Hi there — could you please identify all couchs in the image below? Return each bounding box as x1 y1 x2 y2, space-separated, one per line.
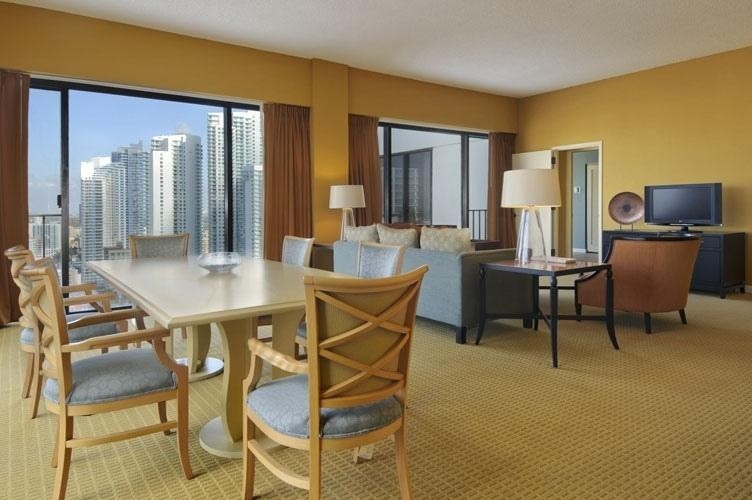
573 230 703 335
333 235 534 343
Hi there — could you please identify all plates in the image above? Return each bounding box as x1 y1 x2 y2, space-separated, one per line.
608 192 644 225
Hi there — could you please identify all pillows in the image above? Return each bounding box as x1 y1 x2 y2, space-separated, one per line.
343 225 379 241
418 226 470 251
377 223 418 247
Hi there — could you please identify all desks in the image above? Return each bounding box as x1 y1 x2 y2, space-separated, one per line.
475 258 621 370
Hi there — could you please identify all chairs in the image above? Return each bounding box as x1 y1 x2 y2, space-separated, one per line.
127 233 191 343
252 234 316 360
5 242 145 422
291 240 408 361
238 262 429 500
32 259 193 500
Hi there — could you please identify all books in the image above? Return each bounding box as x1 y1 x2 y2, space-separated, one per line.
530 254 577 265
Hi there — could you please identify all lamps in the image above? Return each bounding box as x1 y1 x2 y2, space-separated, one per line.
327 184 367 238
499 169 562 263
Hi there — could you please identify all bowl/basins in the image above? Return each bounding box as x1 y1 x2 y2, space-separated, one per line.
197 252 241 273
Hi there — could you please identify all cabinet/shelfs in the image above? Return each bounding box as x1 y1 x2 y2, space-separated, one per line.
602 228 746 298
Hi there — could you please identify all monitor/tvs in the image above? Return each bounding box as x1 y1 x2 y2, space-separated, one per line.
643 183 722 233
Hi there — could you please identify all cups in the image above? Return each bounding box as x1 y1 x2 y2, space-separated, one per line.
524 248 532 263
